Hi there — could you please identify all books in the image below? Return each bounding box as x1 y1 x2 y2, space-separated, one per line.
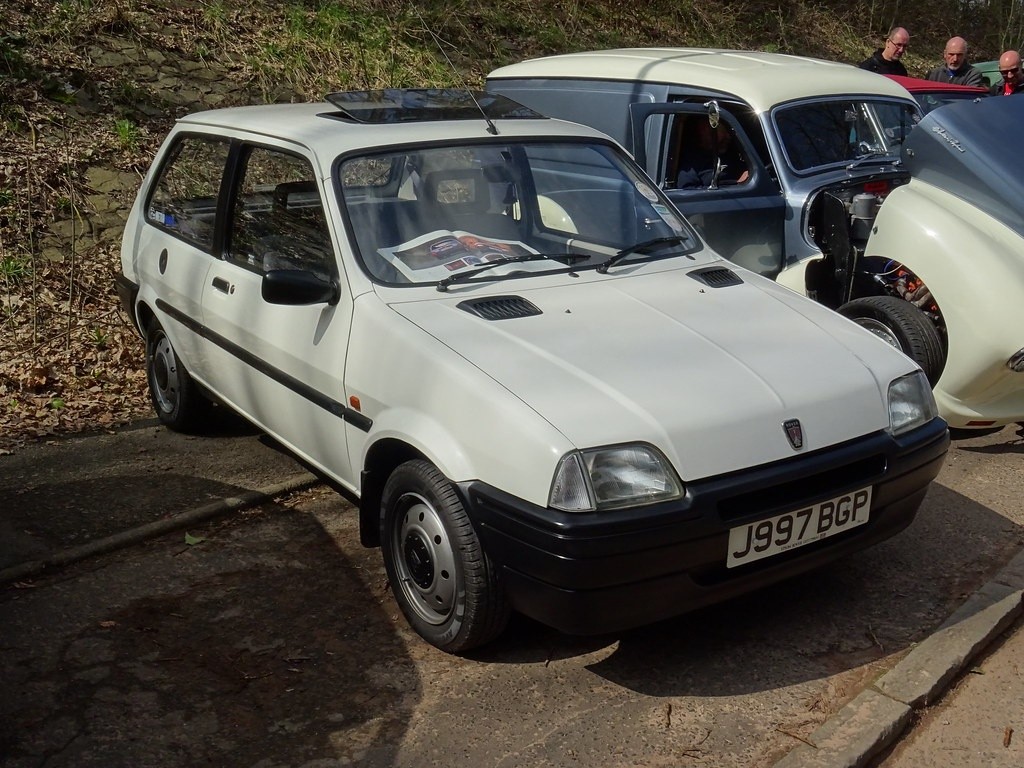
377 229 572 283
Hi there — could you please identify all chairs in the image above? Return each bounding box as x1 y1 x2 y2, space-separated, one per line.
421 168 522 247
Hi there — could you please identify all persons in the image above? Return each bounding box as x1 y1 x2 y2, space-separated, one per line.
778 105 874 170
854 27 910 77
665 104 774 189
917 36 985 117
989 50 1024 96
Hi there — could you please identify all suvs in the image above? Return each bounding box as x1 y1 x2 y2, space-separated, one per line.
122 87 952 655
482 49 1024 431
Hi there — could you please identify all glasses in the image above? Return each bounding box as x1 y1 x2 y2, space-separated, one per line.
888 38 909 50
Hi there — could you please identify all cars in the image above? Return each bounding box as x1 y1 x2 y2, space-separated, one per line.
853 75 994 194
